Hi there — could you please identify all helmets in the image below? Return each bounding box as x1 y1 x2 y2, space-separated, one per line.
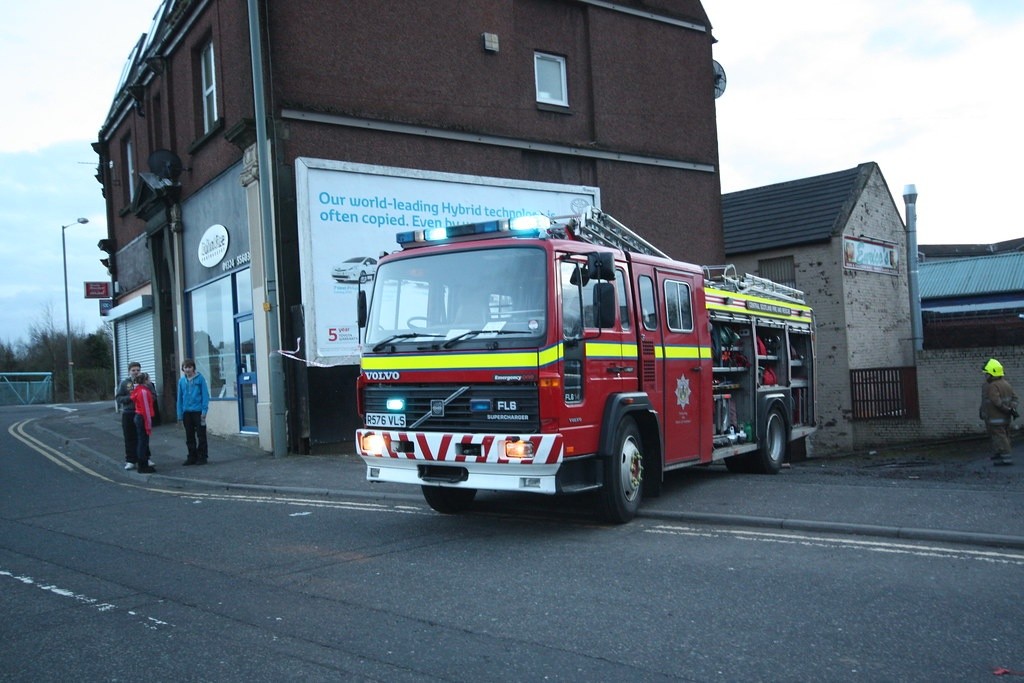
983 359 1006 377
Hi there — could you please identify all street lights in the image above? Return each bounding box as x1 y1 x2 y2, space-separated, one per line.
61 217 91 402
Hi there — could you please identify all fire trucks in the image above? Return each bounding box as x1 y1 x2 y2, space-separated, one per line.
350 203 820 526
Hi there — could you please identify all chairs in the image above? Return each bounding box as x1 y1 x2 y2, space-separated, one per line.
454 280 491 327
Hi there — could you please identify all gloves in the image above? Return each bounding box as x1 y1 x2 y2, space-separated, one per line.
1010 408 1020 420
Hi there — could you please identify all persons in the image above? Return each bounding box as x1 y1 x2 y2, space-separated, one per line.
979 357 1020 466
131 371 158 473
177 358 211 466
114 361 156 470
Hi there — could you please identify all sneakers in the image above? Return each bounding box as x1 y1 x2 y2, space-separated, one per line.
147 460 156 467
124 463 136 469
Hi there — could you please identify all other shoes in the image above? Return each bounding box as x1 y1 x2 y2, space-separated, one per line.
196 459 207 464
138 466 155 473
991 454 1001 460
183 460 198 465
993 460 1012 465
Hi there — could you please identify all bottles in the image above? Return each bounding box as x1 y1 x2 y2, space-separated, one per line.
201 418 205 426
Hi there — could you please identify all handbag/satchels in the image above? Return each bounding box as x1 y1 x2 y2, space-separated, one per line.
1008 415 1021 430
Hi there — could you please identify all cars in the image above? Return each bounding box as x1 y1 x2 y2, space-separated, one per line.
330 256 380 284
